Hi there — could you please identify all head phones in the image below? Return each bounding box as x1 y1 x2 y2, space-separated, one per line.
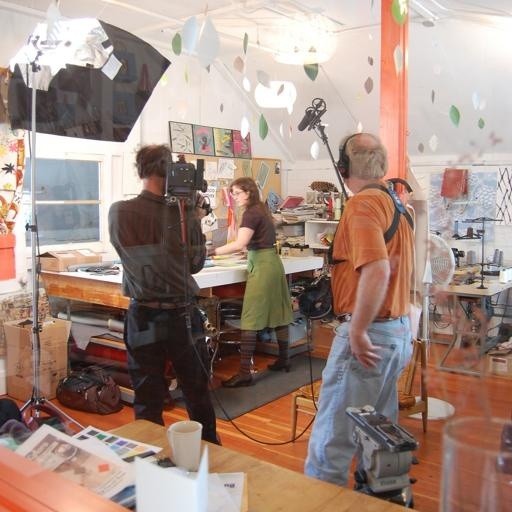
338 133 360 178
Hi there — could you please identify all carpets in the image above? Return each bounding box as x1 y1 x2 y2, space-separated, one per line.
170 354 332 424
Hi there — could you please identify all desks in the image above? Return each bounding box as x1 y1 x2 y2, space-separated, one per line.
436 266 510 383
39 253 325 403
103 414 415 511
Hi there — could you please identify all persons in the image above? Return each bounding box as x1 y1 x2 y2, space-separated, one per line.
204 177 294 388
108 144 227 449
301 132 414 490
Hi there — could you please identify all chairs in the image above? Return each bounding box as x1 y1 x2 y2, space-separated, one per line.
289 336 431 449
206 299 243 379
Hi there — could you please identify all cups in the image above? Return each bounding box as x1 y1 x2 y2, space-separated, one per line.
439 416 512 511
168 421 203 472
465 250 476 265
492 248 503 267
467 227 473 238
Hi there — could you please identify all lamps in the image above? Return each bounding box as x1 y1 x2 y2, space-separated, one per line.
5 14 171 436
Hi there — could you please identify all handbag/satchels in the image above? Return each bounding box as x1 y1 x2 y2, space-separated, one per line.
55 364 124 416
298 273 334 320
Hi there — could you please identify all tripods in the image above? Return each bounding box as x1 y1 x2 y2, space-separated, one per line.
20 122 83 432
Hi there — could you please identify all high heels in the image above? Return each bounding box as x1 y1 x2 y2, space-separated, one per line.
221 371 257 388
266 360 292 373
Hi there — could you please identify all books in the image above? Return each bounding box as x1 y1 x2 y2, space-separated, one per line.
278 194 304 209
277 204 329 247
16 416 168 509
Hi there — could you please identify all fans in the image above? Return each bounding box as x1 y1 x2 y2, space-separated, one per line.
411 229 461 423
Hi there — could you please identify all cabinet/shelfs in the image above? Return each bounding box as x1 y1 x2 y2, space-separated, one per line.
305 220 338 249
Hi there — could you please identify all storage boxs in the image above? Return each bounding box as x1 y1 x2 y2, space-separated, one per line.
279 223 304 238
3 316 73 406
485 346 512 379
36 247 103 273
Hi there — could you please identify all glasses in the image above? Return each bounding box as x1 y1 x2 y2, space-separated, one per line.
231 189 244 196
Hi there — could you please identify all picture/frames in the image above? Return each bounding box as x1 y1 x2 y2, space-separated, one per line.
168 120 254 160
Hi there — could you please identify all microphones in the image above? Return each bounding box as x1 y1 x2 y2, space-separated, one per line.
298 111 316 131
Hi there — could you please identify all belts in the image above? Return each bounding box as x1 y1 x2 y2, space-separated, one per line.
129 297 191 310
337 311 399 323
